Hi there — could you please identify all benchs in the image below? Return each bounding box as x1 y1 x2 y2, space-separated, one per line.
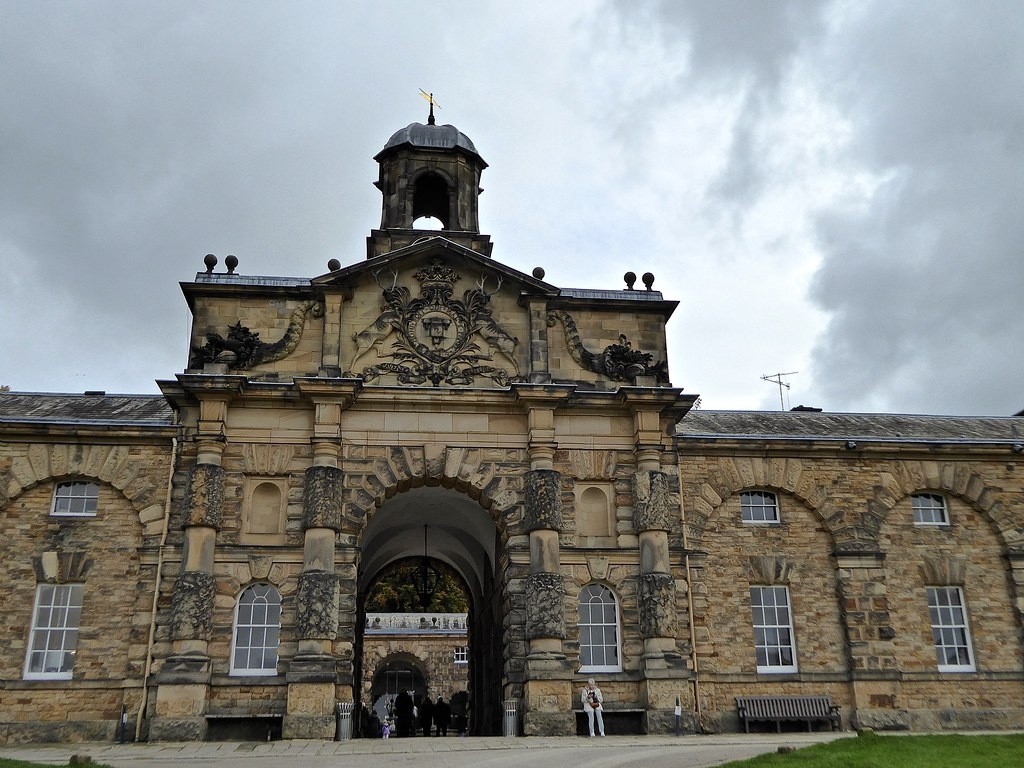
734 695 843 733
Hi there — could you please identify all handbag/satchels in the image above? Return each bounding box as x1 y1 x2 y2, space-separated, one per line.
446 715 451 724
416 718 420 726
587 692 599 708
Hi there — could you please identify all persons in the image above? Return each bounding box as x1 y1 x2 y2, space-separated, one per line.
382 715 391 738
581 678 605 737
419 697 435 737
395 688 414 738
433 696 451 737
369 709 381 737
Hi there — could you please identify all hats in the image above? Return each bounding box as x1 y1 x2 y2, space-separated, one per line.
372 710 377 714
398 688 406 693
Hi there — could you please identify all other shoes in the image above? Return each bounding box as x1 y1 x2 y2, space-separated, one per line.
591 733 595 737
601 731 605 737
